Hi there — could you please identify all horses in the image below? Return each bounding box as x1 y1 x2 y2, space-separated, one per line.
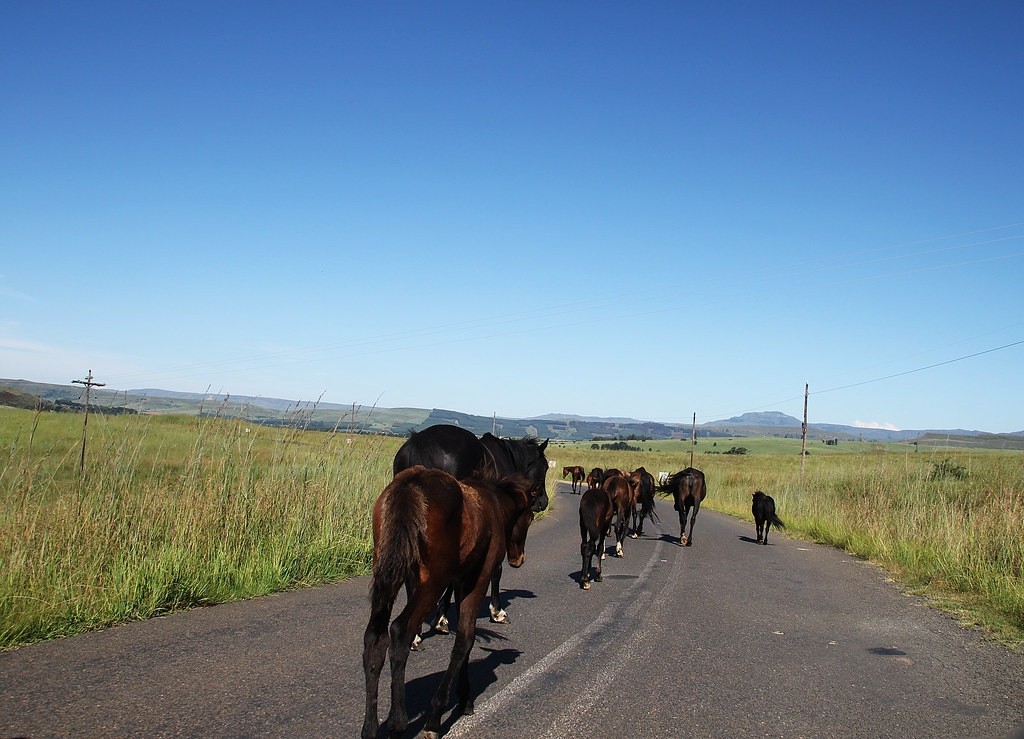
562 466 661 590
655 467 706 547
361 424 553 739
751 491 787 546
